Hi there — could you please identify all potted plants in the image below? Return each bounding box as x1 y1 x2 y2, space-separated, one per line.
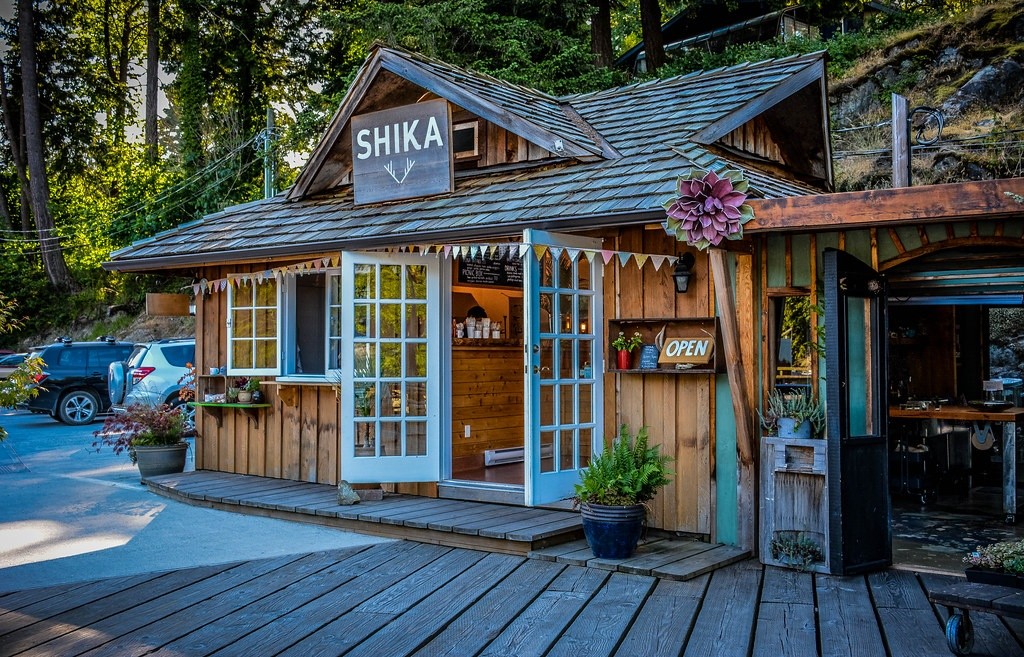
753 386 826 438
134 441 190 484
247 379 266 404
327 352 390 490
573 425 677 559
236 377 252 403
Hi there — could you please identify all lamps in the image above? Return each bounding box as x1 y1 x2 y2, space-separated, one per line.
484 444 553 466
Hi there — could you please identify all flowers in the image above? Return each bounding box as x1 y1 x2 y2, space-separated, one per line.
91 403 199 466
612 331 643 353
660 168 755 251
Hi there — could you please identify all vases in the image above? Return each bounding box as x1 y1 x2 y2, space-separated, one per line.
617 349 631 370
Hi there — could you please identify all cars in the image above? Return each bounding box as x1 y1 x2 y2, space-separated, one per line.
0 352 32 380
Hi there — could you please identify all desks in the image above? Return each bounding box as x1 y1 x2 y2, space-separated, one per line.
889 403 1023 523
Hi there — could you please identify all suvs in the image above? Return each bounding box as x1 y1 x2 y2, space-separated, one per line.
23 335 134 424
107 336 195 437
